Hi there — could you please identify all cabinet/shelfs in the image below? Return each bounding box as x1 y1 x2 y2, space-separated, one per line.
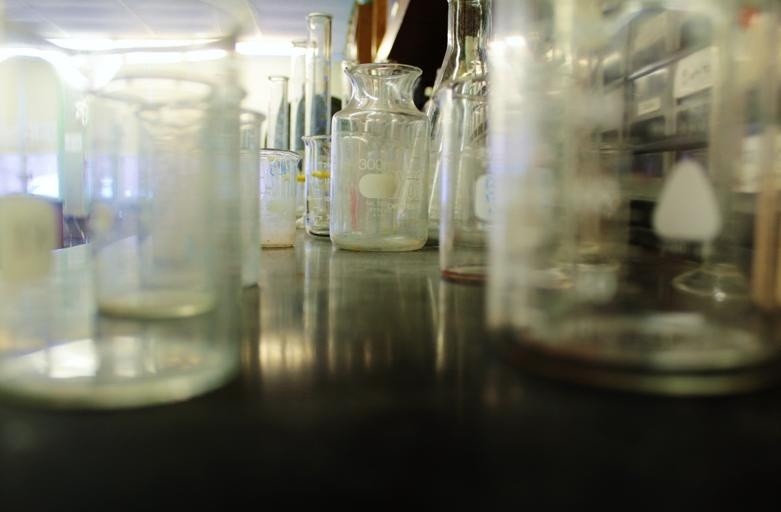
329 60 432 252
390 0 488 244
302 13 334 239
266 75 289 152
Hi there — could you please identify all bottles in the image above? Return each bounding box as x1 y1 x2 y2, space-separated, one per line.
329 60 432 252
390 0 488 244
302 13 334 239
483 1 779 399
266 75 289 152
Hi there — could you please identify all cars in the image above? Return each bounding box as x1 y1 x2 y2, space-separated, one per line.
0 0 260 412
129 105 267 292
299 134 371 235
440 89 575 288
236 148 304 249
79 73 225 322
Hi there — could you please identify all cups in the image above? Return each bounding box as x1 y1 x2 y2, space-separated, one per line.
440 89 575 288
129 105 267 292
236 148 304 249
299 134 371 235
0 0 260 412
79 73 225 322
